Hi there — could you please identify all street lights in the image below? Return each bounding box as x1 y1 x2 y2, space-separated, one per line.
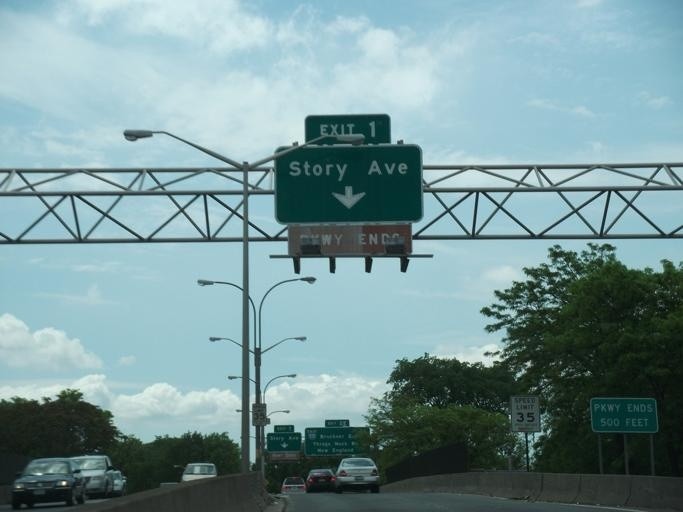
233 408 291 466
228 374 298 462
196 276 318 471
122 129 367 472
240 435 266 463
209 335 307 475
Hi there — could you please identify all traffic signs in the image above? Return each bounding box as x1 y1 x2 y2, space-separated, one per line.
591 397 658 433
275 147 423 222
274 425 293 432
265 450 300 462
288 223 412 256
511 396 540 432
305 427 370 457
305 115 390 144
267 433 301 451
324 419 349 428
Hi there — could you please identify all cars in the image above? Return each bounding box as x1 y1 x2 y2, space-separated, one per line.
180 462 218 483
280 476 306 495
304 468 334 491
70 453 115 499
7 457 86 510
113 470 127 497
334 458 381 493
